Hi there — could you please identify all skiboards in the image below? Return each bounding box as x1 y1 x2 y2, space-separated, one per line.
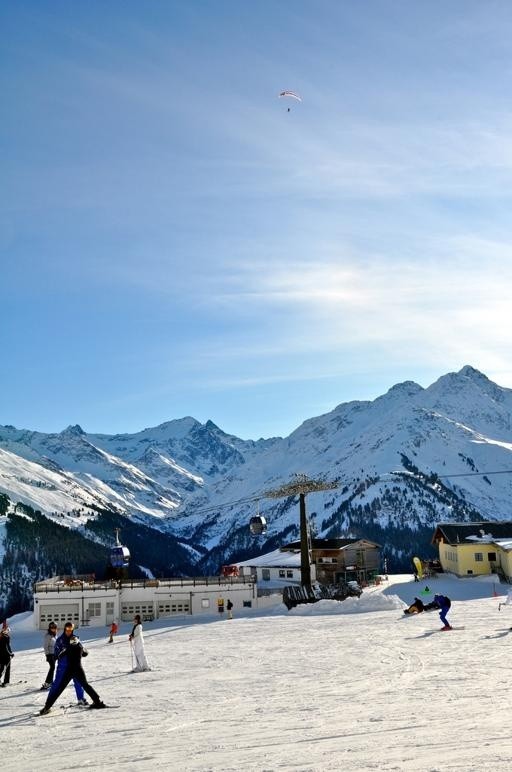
113 670 160 674
28 683 120 717
1 681 27 686
426 626 464 631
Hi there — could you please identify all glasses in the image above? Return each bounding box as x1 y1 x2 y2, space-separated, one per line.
48 624 57 628
65 627 73 631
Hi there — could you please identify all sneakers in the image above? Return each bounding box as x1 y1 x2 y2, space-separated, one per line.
41 682 53 689
39 708 49 714
442 625 452 631
78 699 88 706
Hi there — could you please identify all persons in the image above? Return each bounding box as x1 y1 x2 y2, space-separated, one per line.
109 620 118 643
403 593 453 630
503 587 512 630
216 595 234 619
0 624 14 688
39 621 108 714
129 614 151 672
414 569 419 582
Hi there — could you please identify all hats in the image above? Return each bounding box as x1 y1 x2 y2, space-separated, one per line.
2 626 11 634
134 615 140 621
